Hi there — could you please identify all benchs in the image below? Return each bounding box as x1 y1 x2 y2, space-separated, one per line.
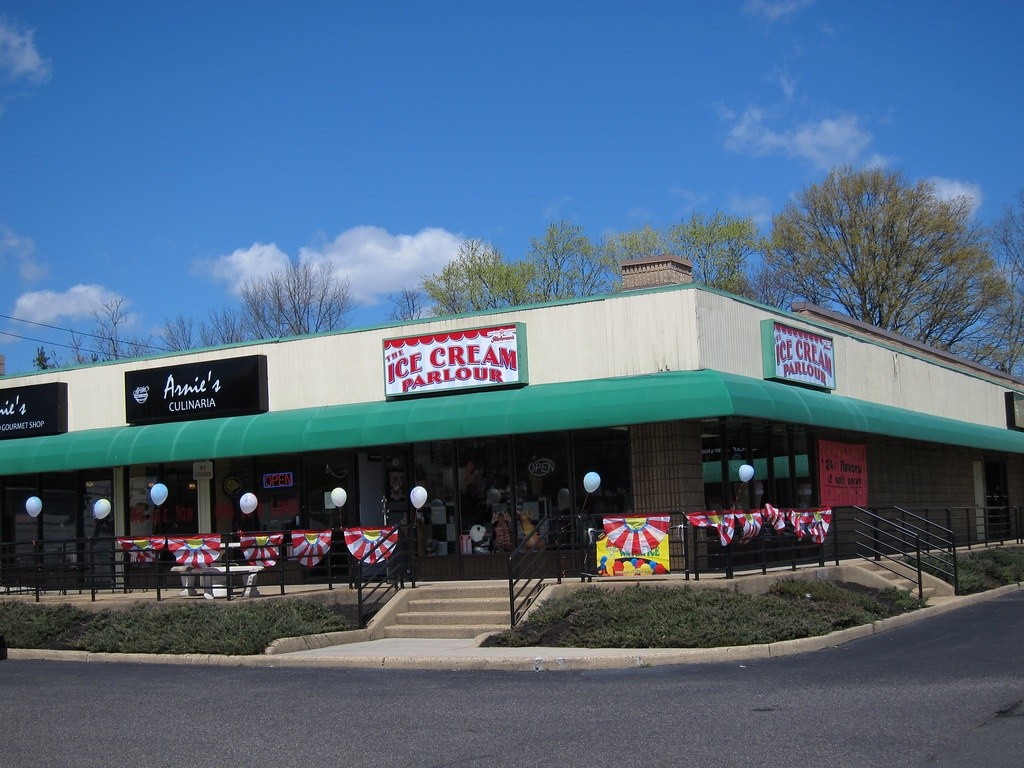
170 566 266 598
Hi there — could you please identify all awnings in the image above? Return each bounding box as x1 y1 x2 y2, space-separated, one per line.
0 368 1024 475
